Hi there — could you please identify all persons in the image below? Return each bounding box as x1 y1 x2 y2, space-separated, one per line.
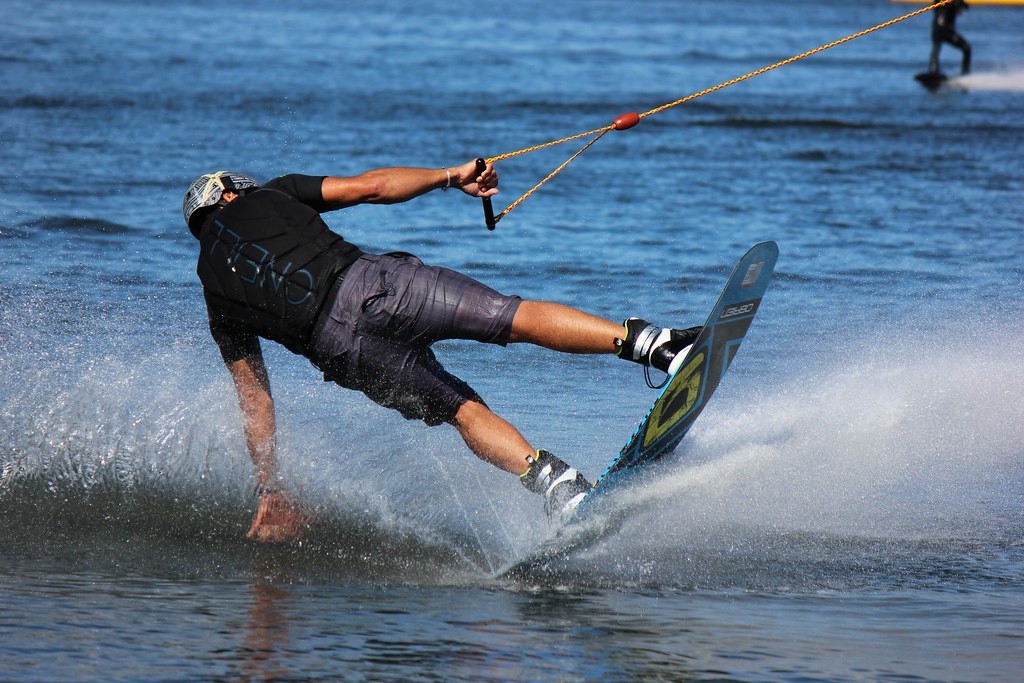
928 0 973 76
179 158 705 548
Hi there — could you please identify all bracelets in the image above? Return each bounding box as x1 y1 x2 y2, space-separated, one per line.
440 167 450 192
254 485 281 498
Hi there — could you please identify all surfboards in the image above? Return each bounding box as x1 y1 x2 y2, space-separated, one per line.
914 72 969 95
493 240 780 579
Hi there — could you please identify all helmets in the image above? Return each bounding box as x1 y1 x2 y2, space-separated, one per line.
181 172 260 239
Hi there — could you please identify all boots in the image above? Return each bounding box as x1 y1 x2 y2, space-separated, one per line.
519 449 594 539
615 316 701 376
